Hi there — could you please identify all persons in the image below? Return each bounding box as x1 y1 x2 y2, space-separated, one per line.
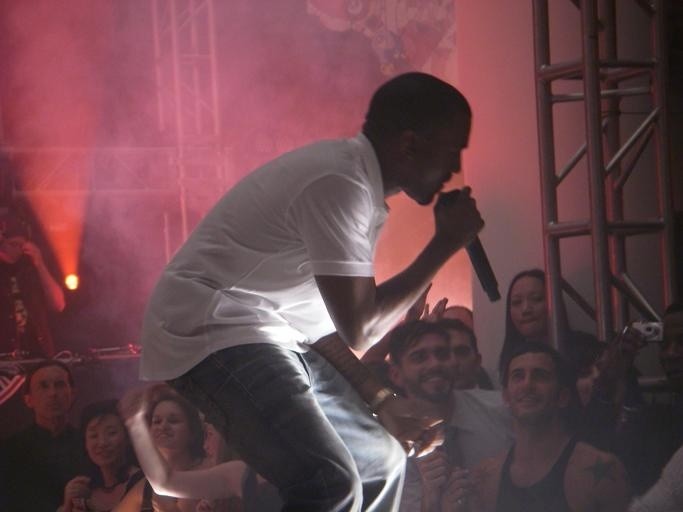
136 73 472 512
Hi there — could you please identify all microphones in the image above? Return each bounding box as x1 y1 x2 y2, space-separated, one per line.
443 190 500 304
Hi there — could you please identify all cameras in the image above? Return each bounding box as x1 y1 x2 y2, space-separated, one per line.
631 321 663 343
